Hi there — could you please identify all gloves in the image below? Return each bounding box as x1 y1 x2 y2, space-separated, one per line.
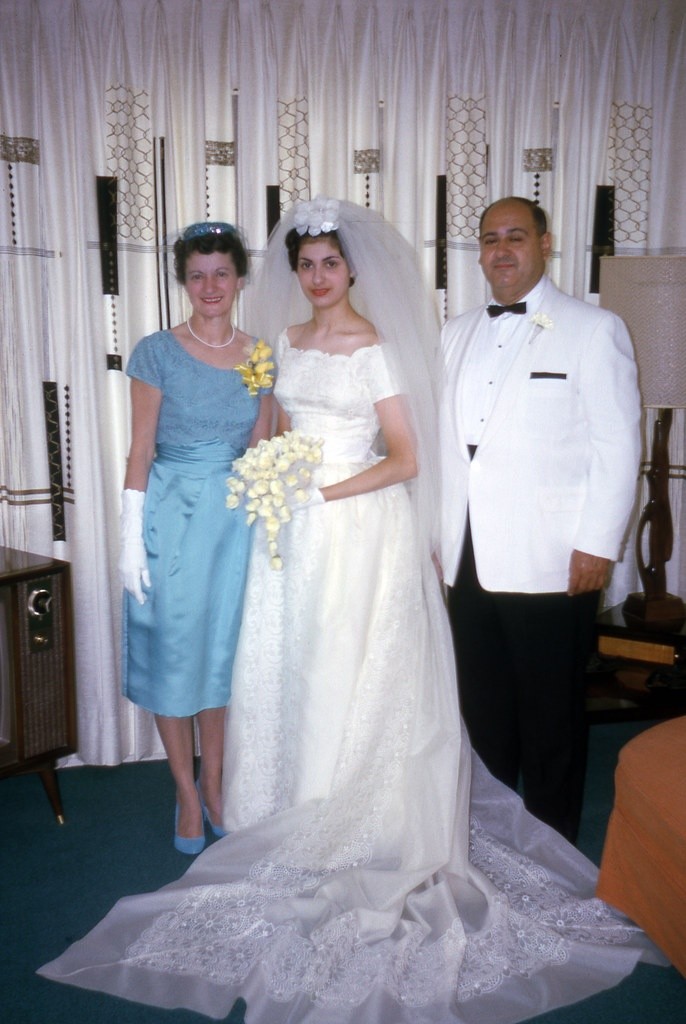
116 489 152 605
289 487 325 510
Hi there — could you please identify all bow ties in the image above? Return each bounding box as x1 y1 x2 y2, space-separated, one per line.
487 302 526 318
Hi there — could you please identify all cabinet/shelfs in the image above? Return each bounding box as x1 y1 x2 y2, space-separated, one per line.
0 545 78 826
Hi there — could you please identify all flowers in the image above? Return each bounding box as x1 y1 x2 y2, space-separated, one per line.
225 430 324 572
233 339 274 398
294 198 341 237
530 313 553 331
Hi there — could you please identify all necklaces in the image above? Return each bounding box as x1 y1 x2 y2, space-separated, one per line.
185 315 235 347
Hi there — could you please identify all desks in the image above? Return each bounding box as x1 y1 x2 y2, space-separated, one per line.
584 600 686 725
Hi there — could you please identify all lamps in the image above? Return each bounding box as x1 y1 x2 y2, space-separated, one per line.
600 255 686 629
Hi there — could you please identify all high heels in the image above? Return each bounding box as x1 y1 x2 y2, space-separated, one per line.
196 779 226 838
174 801 205 855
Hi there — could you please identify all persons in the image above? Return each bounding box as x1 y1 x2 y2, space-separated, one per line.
118 196 644 869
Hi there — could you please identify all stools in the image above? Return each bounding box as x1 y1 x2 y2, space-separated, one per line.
595 716 686 980
